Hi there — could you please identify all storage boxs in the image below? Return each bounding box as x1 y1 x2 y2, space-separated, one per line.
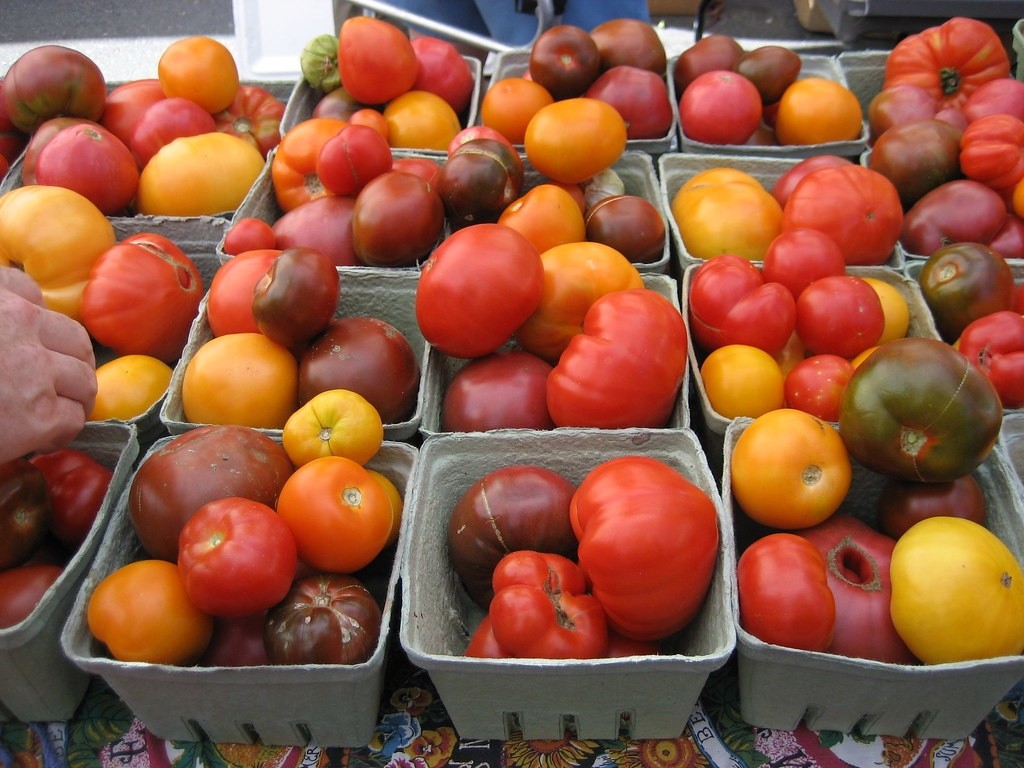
161 269 431 439
413 272 691 434
998 411 1024 500
0 80 296 218
0 422 139 722
95 215 231 469
278 57 482 157
659 151 904 284
856 147 1024 267
398 427 738 742
681 264 945 489
474 50 678 177
904 258 1024 417
441 149 671 274
59 435 417 749
722 416 1024 742
665 53 870 164
212 150 449 272
834 48 1014 150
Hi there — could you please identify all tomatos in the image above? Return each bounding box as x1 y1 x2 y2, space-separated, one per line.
1 7 1024 676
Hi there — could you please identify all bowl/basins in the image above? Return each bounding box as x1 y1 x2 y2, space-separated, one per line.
1 48 1024 745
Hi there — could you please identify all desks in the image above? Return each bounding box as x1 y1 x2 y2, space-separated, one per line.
0 647 1024 768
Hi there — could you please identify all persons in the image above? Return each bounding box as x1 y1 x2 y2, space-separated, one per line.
0 265 99 469
